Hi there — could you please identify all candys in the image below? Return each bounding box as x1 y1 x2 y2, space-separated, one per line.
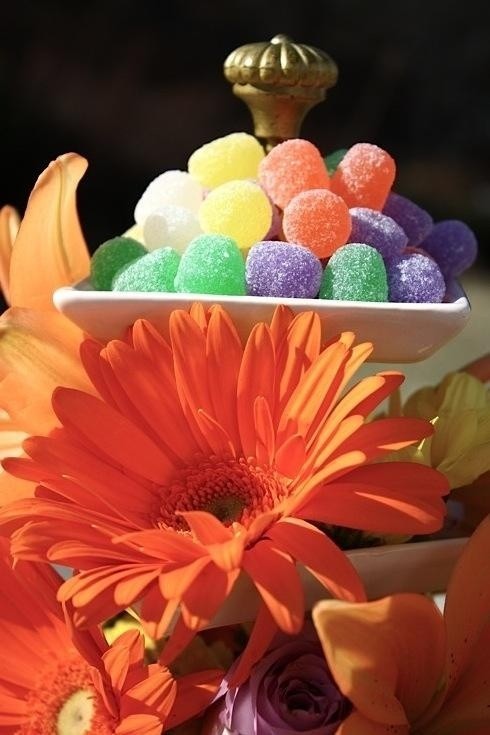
89 131 479 303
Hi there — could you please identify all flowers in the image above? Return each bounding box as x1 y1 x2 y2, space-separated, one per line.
0 146 490 735
